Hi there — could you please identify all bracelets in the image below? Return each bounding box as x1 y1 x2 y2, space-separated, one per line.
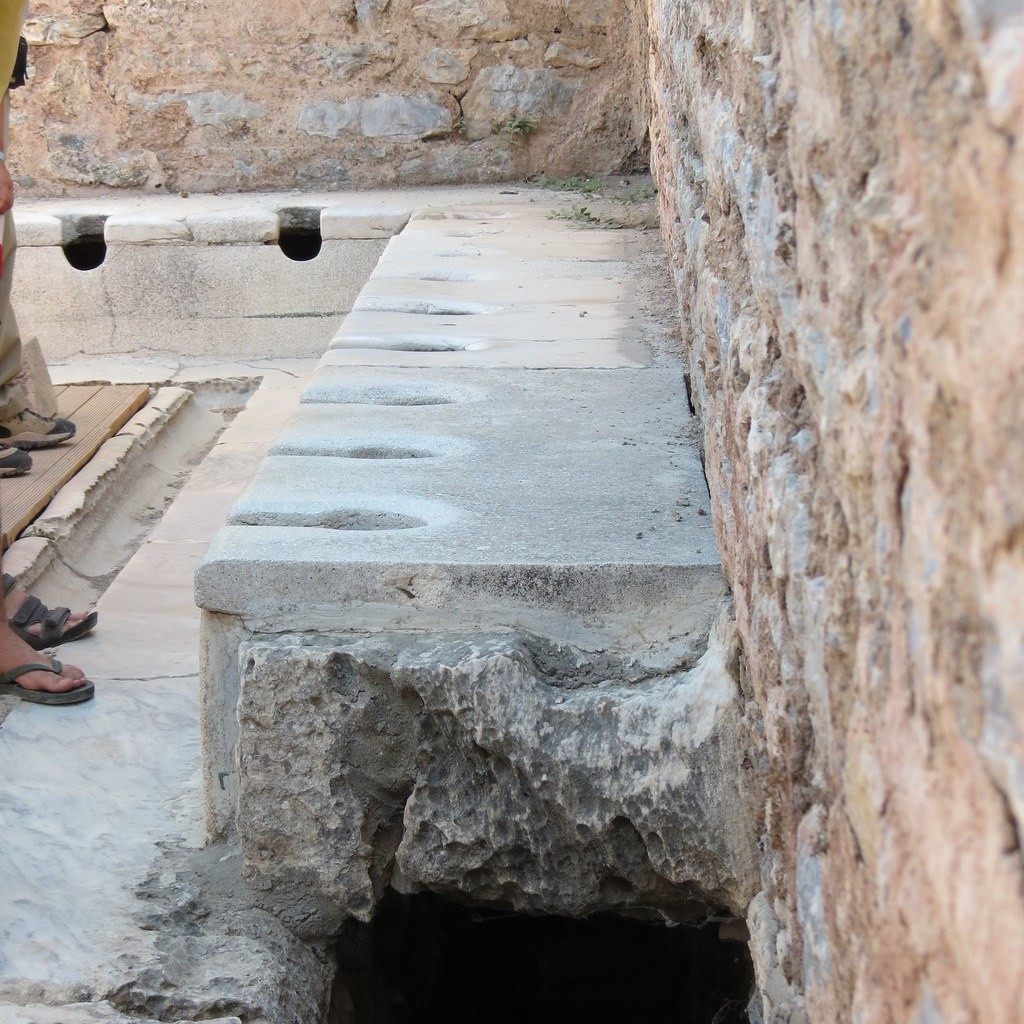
0 152 6 161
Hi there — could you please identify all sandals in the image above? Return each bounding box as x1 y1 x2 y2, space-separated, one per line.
0 575 98 651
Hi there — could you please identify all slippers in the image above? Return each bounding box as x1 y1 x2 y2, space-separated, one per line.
0 655 94 705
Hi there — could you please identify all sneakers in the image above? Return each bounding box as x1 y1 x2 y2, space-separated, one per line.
0 406 77 449
0 442 33 478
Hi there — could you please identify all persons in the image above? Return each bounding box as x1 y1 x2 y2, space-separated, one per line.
0 570 98 704
0 1 77 478
